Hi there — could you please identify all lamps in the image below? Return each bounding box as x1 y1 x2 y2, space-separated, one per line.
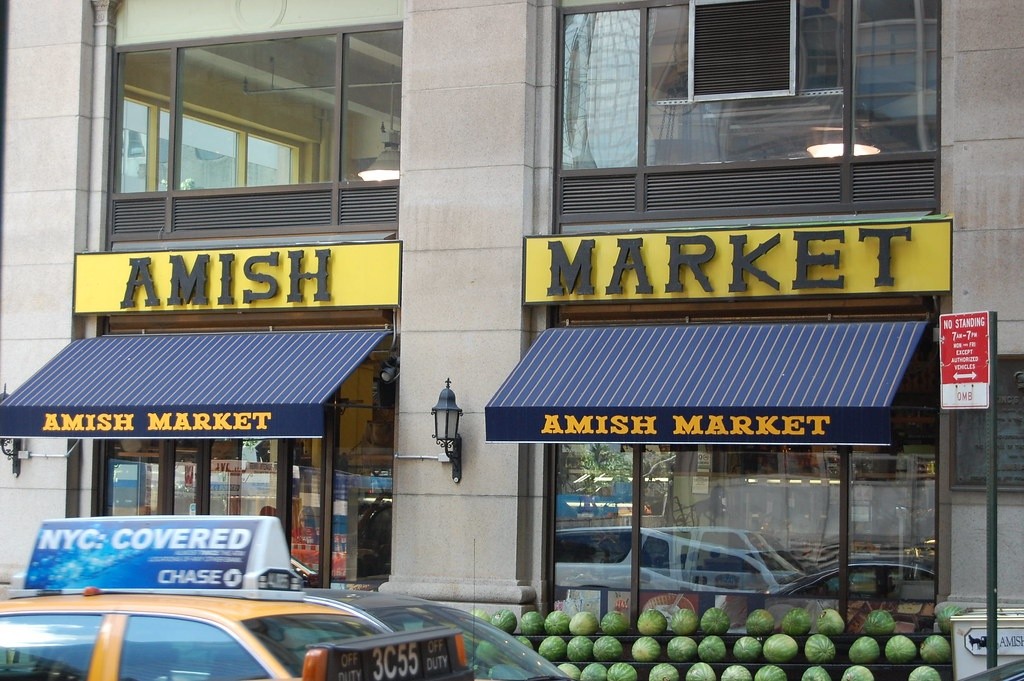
430 377 463 483
357 85 400 180
380 354 400 383
0 383 21 478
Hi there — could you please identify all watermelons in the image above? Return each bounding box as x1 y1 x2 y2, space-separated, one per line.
451 606 966 681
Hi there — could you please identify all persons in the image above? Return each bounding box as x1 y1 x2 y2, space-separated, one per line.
259 506 276 516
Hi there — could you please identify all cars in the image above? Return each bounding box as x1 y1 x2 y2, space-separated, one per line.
0 515 577 681
553 524 937 598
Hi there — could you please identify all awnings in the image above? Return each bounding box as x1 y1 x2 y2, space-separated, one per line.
0 328 390 439
485 320 935 448
